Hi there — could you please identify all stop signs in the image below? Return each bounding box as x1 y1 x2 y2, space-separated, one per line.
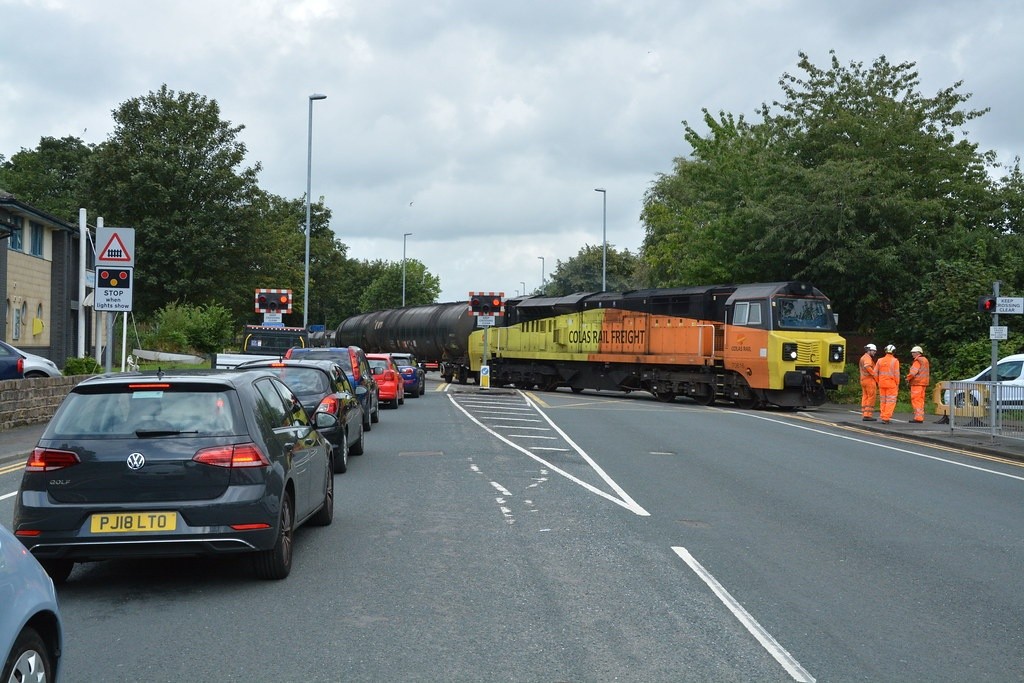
95 266 133 313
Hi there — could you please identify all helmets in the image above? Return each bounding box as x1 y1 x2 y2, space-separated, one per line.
910 346 923 353
864 344 877 351
884 344 896 353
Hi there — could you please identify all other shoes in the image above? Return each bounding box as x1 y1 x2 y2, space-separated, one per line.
909 419 923 423
881 419 893 424
862 416 877 421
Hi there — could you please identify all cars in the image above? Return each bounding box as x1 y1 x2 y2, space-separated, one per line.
944 354 1024 410
363 352 406 409
392 353 425 398
0 368 337 683
0 340 62 381
235 360 368 474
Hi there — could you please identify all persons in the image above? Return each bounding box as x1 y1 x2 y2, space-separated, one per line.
859 344 877 421
873 344 900 424
905 346 929 423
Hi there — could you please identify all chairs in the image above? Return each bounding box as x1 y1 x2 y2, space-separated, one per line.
131 398 174 430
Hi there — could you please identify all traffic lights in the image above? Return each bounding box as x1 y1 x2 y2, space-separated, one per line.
255 288 292 314
978 296 996 314
468 291 505 317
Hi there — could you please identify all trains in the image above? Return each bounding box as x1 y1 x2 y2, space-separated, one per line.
308 281 850 409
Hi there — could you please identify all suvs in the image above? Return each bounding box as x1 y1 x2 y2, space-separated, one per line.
284 346 384 431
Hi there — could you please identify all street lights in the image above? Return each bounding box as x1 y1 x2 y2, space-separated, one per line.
402 233 412 307
538 256 544 295
595 187 607 293
515 282 525 298
304 94 327 328
